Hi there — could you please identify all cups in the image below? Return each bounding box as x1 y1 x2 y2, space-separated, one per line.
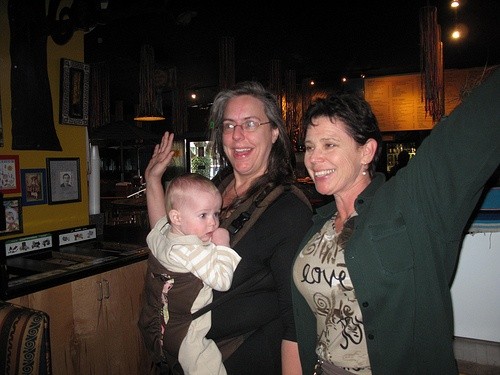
90 147 100 214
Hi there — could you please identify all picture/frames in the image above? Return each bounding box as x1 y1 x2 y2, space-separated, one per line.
0 156 22 194
21 168 48 205
0 196 24 236
46 157 81 205
60 58 91 127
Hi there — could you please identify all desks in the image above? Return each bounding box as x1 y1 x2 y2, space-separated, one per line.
101 182 148 235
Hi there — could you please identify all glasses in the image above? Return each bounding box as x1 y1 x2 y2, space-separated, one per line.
219 121 273 133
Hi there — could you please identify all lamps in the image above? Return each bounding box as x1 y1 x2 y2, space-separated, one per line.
134 84 166 122
46 1 110 46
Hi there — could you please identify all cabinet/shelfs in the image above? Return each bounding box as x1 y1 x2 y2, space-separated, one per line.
0 226 147 375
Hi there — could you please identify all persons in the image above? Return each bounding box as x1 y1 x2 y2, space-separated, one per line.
61 172 70 187
292 67 500 375
136 173 243 375
145 86 313 375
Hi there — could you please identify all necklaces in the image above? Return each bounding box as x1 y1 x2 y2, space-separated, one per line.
233 179 239 197
